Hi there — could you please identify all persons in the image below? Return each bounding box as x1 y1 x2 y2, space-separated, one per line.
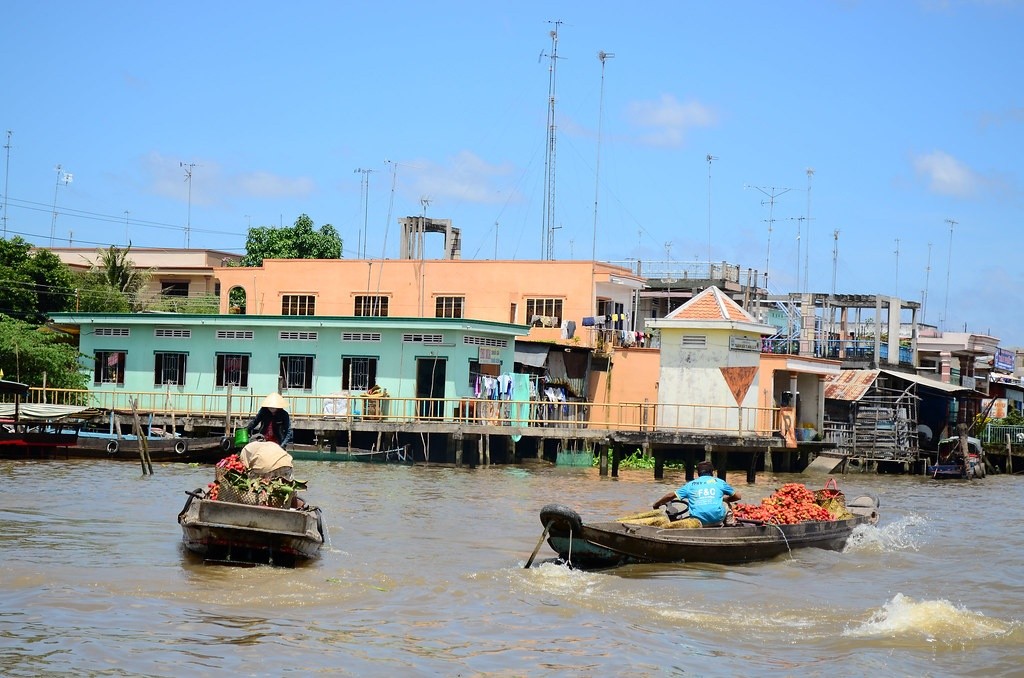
247 392 292 449
238 434 298 508
652 461 741 527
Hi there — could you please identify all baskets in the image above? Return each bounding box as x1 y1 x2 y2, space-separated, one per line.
814 478 846 506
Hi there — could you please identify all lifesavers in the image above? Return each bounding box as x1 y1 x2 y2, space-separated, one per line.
106 439 120 454
185 488 202 509
220 437 233 453
540 503 583 534
174 439 188 455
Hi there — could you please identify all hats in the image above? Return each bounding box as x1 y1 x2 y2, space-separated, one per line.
697 461 715 471
261 392 289 408
250 433 266 442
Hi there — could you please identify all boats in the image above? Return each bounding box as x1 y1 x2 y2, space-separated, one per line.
178 489 323 569
930 436 984 480
285 443 404 461
539 494 879 568
0 403 235 457
